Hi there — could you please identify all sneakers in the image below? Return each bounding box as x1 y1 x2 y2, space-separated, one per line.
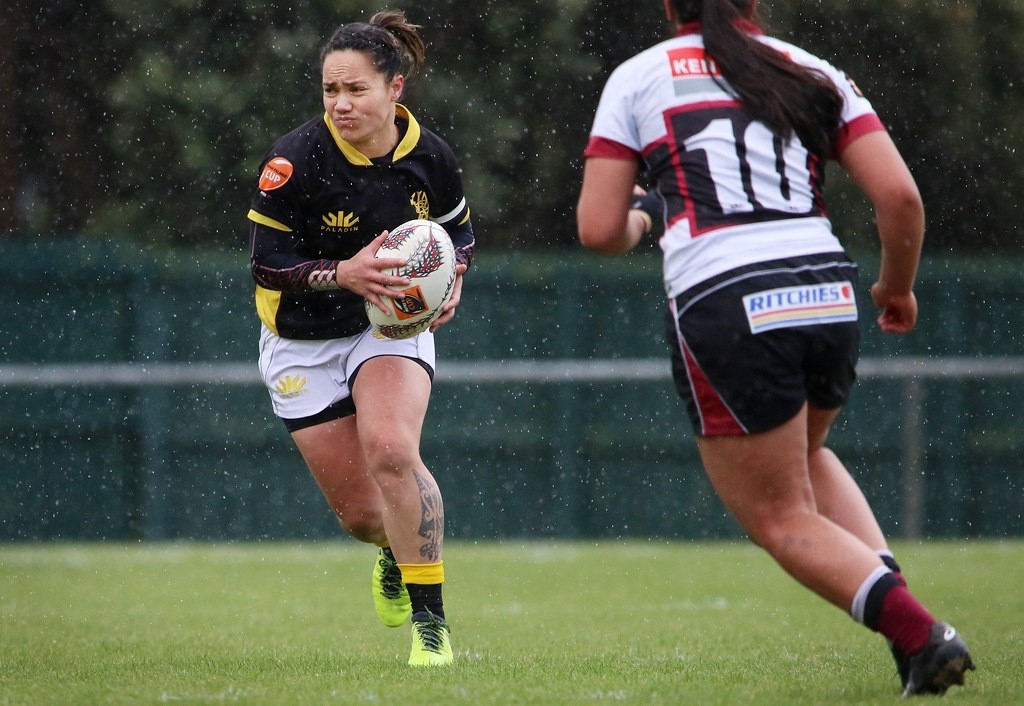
372 547 412 627
408 605 453 668
901 619 976 697
891 645 910 689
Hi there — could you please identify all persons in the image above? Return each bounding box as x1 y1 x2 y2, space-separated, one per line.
247 10 476 668
575 0 978 696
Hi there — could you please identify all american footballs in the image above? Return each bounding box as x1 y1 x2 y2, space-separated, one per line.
364 219 456 340
